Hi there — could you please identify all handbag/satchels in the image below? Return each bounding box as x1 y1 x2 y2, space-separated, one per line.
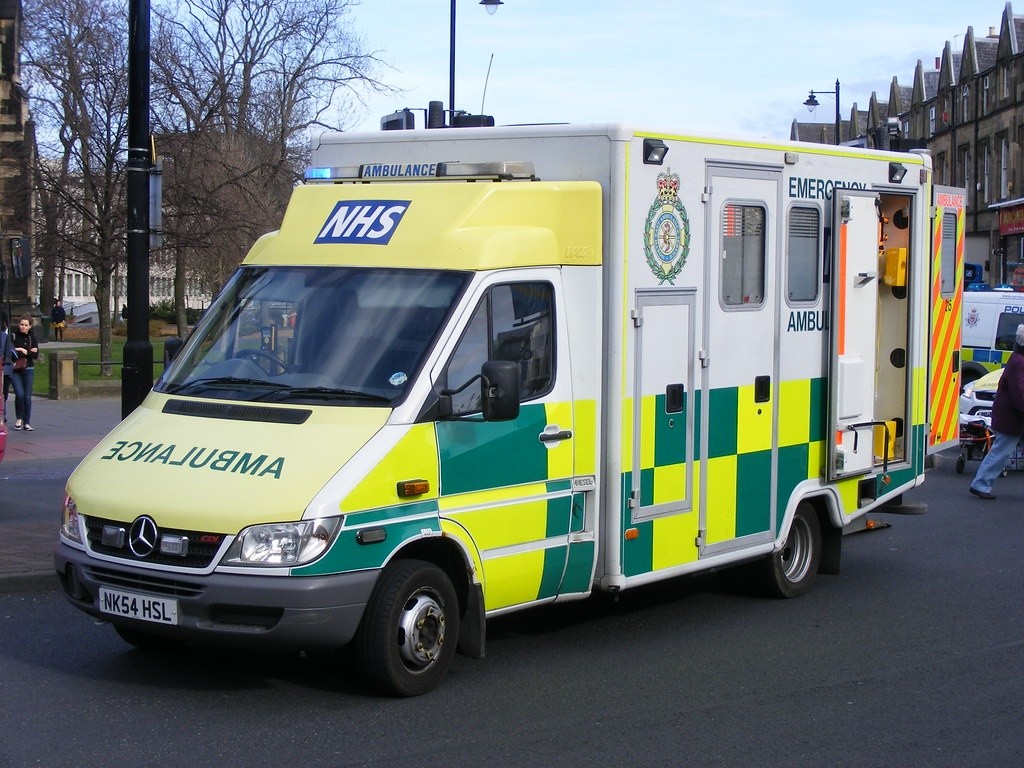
13 357 27 371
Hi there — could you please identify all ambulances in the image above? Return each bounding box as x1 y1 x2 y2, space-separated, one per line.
53 100 968 699
961 282 1024 383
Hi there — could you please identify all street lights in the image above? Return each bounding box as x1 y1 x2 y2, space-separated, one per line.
448 0 503 127
804 79 839 145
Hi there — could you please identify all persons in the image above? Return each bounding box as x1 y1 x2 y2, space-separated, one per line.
969 323 1024 500
0 309 39 431
122 304 127 323
51 300 66 342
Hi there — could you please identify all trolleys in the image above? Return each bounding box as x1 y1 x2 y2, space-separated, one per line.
924 424 1008 477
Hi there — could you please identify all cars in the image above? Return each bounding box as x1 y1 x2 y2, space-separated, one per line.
960 365 1005 448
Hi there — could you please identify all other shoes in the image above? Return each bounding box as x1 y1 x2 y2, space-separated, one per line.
15 422 22 429
4 420 7 424
969 486 997 499
24 424 33 431
